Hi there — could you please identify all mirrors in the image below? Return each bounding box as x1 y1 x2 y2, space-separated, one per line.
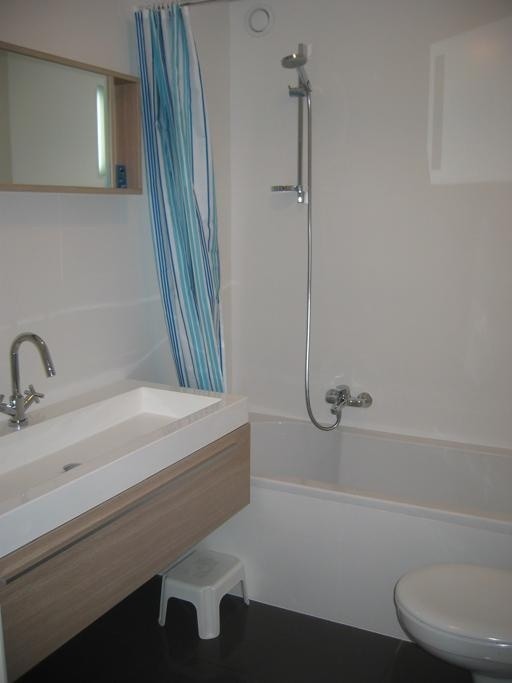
0 37 113 195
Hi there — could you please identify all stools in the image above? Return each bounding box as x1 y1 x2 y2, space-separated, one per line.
155 550 249 640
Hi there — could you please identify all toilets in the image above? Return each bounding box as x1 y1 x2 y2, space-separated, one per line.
392 562 512 683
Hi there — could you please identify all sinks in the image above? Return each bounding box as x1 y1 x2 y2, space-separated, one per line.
0 376 250 560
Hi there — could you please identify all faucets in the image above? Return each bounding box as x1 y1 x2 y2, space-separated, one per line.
10 332 56 426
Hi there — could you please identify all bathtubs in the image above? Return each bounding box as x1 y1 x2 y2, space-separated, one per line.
195 409 512 645
